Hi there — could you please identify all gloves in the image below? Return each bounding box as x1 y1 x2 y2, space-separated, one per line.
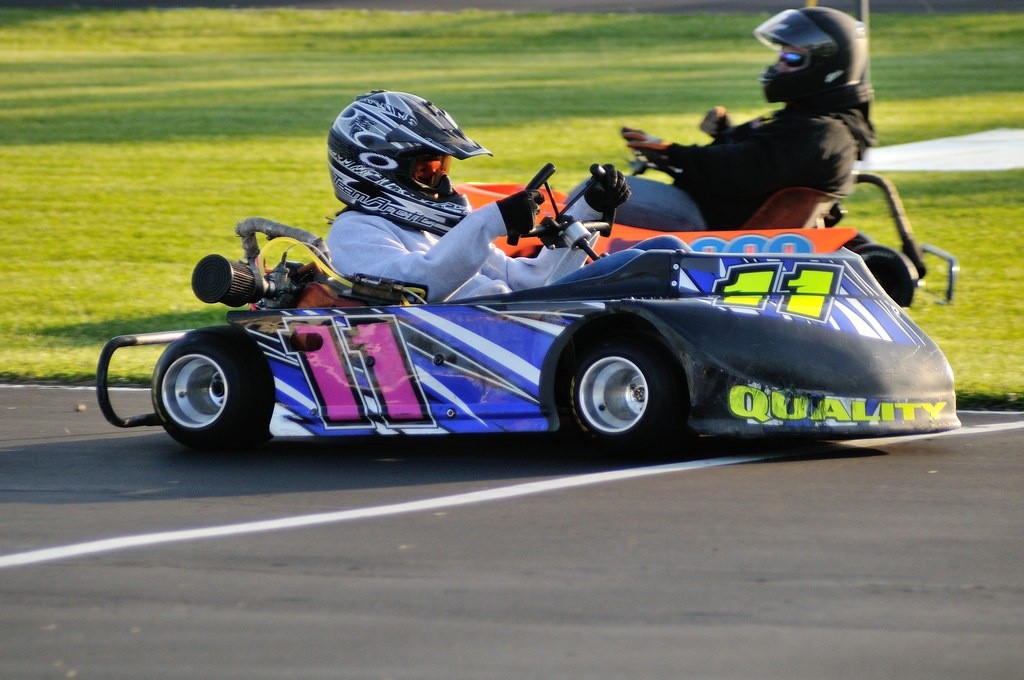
621 127 672 153
701 107 731 138
497 190 546 245
584 163 631 213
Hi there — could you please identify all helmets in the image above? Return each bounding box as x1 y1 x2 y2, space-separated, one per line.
328 89 494 235
762 7 872 113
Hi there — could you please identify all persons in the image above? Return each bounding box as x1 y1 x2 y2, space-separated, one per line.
566 5 870 229
326 90 694 301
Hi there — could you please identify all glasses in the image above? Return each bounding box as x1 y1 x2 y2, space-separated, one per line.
778 52 804 67
409 154 451 186
